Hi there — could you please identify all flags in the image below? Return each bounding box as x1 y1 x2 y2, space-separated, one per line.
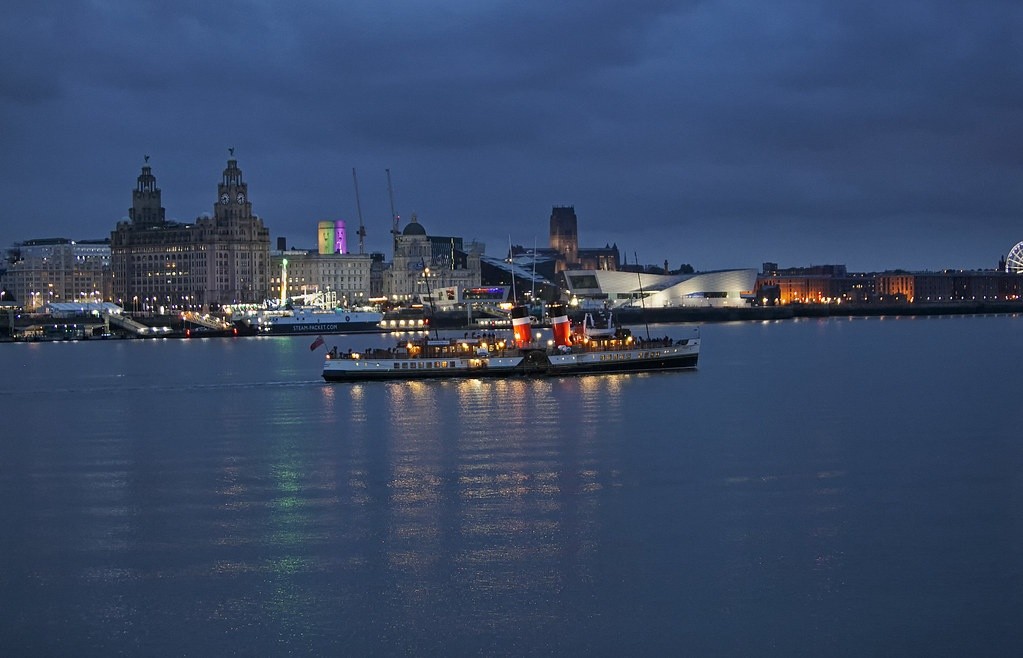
310 335 324 352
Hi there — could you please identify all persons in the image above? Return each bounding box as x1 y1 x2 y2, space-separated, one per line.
464 331 495 340
639 336 673 347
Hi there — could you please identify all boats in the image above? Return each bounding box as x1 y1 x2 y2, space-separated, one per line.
582 308 617 338
231 292 386 335
307 233 702 383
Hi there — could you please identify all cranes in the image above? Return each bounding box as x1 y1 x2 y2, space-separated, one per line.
384 167 400 250
351 166 368 255
